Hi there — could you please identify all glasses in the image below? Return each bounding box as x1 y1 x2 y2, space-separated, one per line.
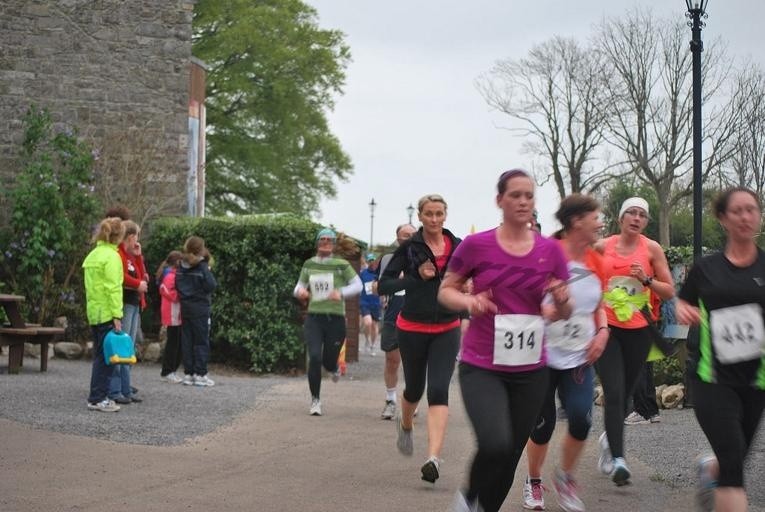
625 211 647 219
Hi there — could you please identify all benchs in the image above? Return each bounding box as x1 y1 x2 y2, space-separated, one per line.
0 295 64 377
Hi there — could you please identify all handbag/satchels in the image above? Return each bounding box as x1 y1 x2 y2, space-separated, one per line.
103 329 137 366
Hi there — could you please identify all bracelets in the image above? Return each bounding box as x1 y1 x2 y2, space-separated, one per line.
599 327 611 332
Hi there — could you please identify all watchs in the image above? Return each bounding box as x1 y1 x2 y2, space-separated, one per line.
642 276 653 287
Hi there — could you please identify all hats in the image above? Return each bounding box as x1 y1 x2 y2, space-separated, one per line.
318 229 338 242
366 255 376 261
618 198 651 221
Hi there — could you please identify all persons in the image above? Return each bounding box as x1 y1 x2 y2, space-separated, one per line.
357 254 382 356
377 194 463 483
293 229 363 416
593 197 675 488
174 236 218 387
106 220 148 404
437 168 574 512
375 224 418 421
82 216 126 413
156 250 183 384
674 188 764 512
521 195 613 512
624 343 660 425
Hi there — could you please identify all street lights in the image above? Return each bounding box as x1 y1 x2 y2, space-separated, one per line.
684 0 709 260
406 203 415 227
367 197 377 252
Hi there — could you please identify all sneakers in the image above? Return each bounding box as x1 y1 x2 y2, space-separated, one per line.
598 432 614 474
612 459 631 486
523 478 544 509
194 373 213 385
126 393 143 401
183 375 195 385
162 373 182 383
624 410 651 426
694 456 715 508
114 395 131 403
88 398 119 412
380 400 397 420
309 400 322 416
397 423 413 455
554 470 583 511
421 457 440 483
